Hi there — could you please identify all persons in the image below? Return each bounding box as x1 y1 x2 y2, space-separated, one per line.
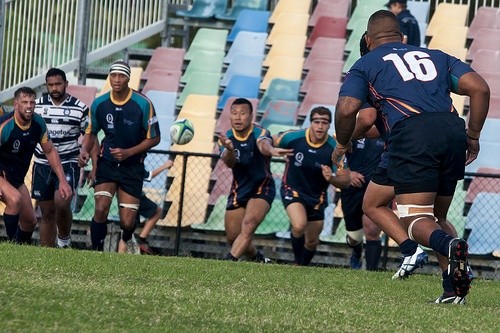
0 87 72 243
272 107 350 265
333 0 491 304
218 98 294 264
32 68 99 249
137 153 173 254
77 60 160 255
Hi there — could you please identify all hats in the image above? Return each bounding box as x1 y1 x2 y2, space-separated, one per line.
109 60 131 78
384 0 406 7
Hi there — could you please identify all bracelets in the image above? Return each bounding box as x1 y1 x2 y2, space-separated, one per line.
466 130 479 140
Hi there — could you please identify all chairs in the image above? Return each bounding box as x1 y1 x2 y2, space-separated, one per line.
0 0 500 257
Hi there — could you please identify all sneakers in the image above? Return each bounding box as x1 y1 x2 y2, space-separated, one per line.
137 240 156 255
447 238 470 299
349 248 362 269
435 293 466 305
392 247 428 281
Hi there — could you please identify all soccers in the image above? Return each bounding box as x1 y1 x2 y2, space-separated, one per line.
170 117 194 145
